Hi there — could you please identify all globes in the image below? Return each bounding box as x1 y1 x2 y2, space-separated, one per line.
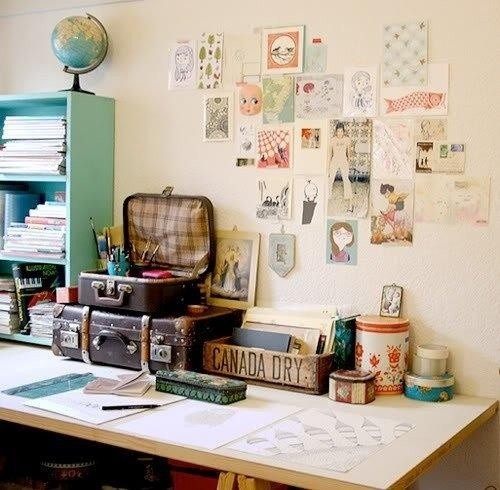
51 12 108 95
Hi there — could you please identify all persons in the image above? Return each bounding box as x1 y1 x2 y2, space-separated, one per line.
220 254 242 293
326 122 356 214
237 83 264 116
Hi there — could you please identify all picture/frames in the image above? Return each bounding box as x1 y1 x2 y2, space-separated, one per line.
259 25 305 76
203 230 260 310
380 285 402 318
202 91 234 142
254 125 294 170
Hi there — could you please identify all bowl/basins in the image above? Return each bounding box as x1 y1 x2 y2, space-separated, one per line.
187 304 209 316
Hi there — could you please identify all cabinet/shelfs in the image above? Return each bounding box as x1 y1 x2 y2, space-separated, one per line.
0 92 114 349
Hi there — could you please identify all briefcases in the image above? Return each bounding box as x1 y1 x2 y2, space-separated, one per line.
77 190 219 311
50 302 243 373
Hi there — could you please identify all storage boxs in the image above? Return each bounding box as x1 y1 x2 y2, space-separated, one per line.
202 331 347 394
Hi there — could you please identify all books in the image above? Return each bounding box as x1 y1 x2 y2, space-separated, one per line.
82 371 152 397
0 113 67 339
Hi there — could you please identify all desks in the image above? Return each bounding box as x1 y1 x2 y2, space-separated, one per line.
0 339 500 490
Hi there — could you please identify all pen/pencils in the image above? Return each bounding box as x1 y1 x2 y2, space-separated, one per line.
102 405 162 410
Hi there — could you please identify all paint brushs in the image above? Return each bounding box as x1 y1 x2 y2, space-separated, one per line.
132 235 161 263
90 217 101 259
105 225 120 263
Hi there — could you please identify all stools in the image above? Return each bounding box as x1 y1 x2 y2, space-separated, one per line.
39 456 96 490
